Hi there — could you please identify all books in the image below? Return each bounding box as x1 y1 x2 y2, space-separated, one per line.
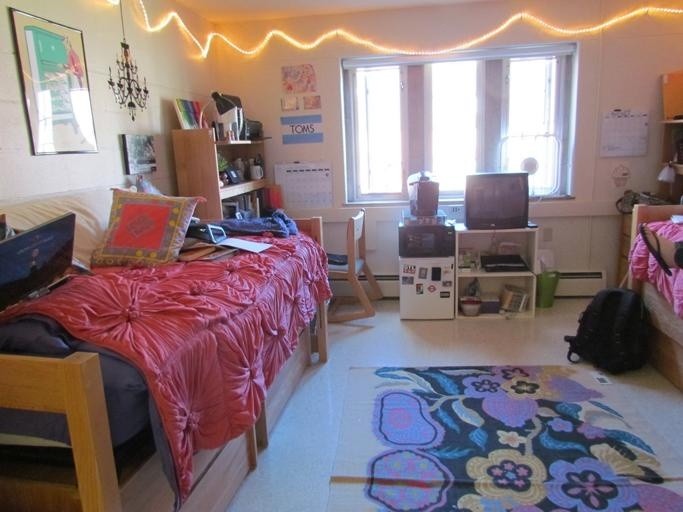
233 195 261 218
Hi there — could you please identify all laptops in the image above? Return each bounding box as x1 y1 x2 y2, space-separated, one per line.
0 210 76 315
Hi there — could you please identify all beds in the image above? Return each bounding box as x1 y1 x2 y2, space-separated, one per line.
0 188 328 512
628 205 683 393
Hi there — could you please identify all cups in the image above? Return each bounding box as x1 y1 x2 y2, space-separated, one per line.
249 165 264 180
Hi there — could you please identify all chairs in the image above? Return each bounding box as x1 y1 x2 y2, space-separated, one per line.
323 207 383 323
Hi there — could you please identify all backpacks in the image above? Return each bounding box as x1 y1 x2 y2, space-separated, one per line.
564 287 653 377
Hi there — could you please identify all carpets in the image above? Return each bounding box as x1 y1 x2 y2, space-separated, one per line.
327 363 683 511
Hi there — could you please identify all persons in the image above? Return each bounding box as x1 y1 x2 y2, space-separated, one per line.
61 37 84 88
638 222 683 276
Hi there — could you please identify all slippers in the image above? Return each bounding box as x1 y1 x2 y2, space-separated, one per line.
639 222 673 276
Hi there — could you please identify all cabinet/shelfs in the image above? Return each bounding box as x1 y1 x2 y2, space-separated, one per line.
173 122 272 218
454 219 539 320
660 116 683 205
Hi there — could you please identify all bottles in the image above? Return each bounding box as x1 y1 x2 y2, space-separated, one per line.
202 119 239 142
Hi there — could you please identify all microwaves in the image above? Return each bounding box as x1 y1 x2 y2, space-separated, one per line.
397 219 455 259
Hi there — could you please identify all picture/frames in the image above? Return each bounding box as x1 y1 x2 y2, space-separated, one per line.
9 4 99 156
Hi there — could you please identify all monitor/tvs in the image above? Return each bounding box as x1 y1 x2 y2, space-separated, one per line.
466 173 529 229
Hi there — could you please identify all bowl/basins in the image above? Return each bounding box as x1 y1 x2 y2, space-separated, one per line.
460 300 481 317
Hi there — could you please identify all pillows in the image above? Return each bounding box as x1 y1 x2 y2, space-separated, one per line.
137 175 199 223
89 188 207 266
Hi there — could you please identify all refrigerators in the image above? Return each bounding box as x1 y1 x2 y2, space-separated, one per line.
398 256 456 322
